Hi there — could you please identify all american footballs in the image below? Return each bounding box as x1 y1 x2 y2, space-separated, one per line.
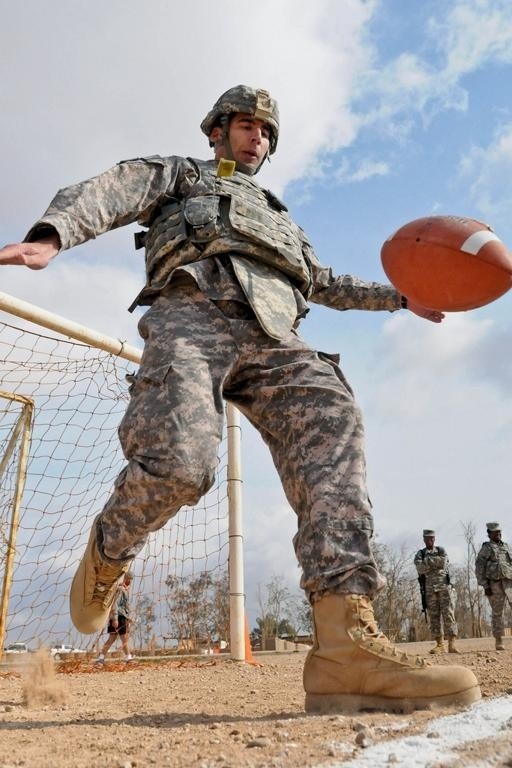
381 216 512 312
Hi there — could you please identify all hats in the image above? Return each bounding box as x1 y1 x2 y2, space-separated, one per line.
423 528 436 536
486 522 501 531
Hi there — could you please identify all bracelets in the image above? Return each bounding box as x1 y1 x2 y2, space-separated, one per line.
401 296 407 309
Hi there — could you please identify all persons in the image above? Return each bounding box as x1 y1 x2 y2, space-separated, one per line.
413 529 462 653
96 571 133 666
0 84 482 713
475 522 512 650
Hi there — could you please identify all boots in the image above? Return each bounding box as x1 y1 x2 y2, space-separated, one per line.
496 635 505 649
303 591 484 713
431 636 445 653
70 513 133 633
449 639 459 651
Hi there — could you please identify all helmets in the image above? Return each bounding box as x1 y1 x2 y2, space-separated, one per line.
200 84 279 154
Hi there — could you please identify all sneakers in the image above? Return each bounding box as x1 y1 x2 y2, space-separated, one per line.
95 658 104 665
120 657 138 663
